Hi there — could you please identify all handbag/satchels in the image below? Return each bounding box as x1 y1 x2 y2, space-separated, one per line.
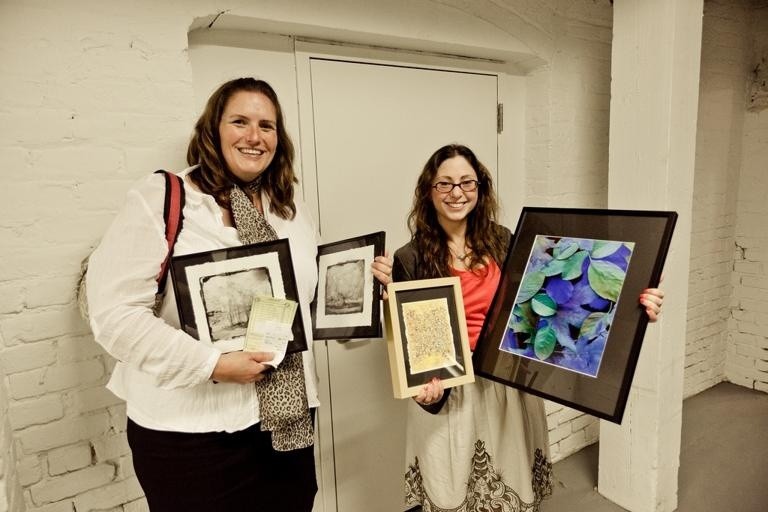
75 245 164 328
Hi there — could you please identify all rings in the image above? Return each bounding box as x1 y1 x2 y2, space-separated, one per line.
422 401 432 407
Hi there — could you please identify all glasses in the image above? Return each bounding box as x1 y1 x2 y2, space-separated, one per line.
431 180 481 193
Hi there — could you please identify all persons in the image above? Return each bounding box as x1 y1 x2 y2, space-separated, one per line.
381 143 669 512
84 74 391 511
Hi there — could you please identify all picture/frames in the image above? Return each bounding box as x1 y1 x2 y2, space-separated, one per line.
311 231 387 340
473 206 679 424
386 275 476 400
168 237 308 385
